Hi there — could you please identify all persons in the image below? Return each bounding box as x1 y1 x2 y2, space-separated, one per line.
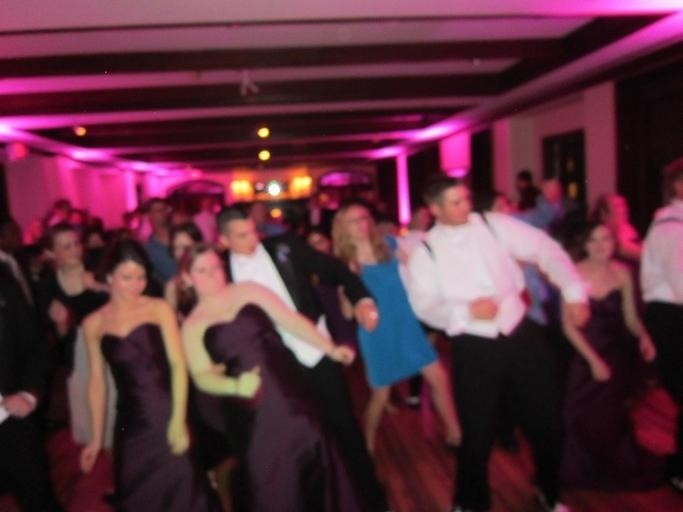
1 158 683 511
